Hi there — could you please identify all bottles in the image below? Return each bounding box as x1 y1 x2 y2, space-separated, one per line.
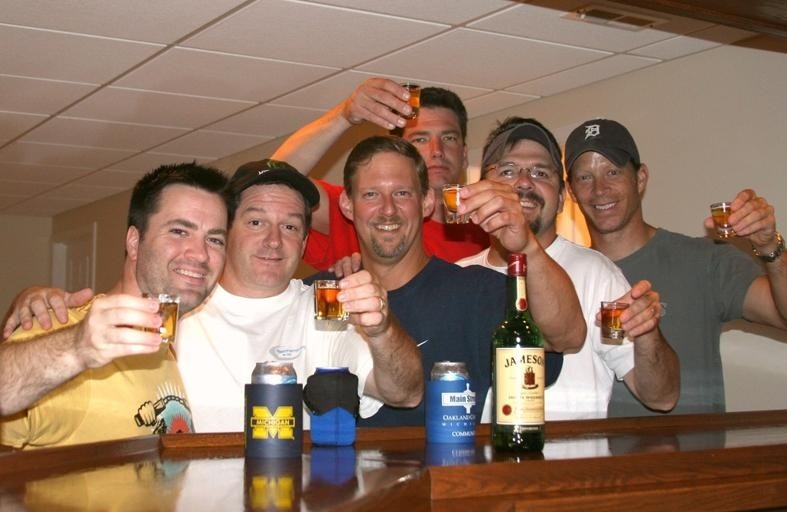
244 363 303 459
309 366 358 446
424 360 478 445
488 253 547 454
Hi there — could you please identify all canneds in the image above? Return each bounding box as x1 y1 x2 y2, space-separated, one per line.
430 361 469 443
251 361 297 458
314 367 350 445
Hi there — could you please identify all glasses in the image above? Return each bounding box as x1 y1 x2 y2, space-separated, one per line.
485 163 552 179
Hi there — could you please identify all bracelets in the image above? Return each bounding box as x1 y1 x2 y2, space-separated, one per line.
751 231 785 263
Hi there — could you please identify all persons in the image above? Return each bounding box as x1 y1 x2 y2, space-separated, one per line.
0 157 426 434
298 134 587 427
450 115 682 424
564 118 787 456
1 162 236 450
270 76 492 273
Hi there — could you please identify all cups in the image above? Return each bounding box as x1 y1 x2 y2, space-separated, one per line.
139 291 179 343
391 82 422 128
441 185 470 226
313 280 350 320
602 300 629 345
710 201 738 238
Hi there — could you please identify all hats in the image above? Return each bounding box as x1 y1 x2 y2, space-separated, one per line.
565 119 639 174
481 123 563 173
229 159 319 208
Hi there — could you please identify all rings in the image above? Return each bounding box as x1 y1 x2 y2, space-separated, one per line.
648 305 656 316
378 296 387 312
